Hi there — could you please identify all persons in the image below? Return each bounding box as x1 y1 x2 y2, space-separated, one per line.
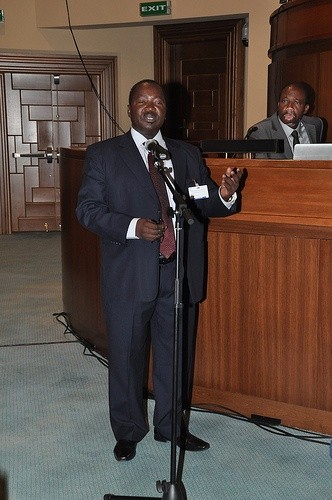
247 82 323 160
77 79 241 460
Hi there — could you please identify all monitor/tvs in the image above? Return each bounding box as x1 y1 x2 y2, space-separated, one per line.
293 144 332 160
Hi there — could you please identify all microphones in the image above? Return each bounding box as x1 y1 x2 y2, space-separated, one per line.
145 139 168 160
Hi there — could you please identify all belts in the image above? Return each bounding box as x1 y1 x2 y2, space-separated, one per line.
158 254 176 264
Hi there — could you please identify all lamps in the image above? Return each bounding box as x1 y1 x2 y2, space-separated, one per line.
241 23 248 47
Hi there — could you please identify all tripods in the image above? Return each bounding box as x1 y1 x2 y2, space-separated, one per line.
104 151 194 500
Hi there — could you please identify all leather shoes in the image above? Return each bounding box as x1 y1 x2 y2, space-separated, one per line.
113 440 137 462
154 427 210 451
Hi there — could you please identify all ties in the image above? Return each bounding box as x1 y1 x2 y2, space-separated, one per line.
291 131 300 151
148 152 176 257
